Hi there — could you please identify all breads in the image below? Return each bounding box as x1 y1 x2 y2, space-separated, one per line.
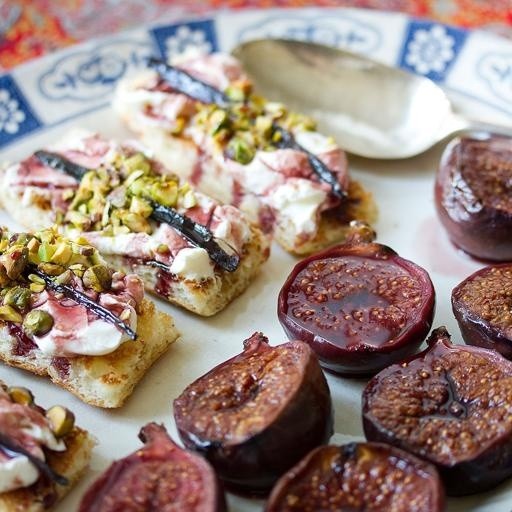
0 379 96 512
0 128 270 319
110 51 377 258
0 227 180 410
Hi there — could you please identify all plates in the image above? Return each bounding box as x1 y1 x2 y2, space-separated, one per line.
0 3 510 510
230 36 511 163
